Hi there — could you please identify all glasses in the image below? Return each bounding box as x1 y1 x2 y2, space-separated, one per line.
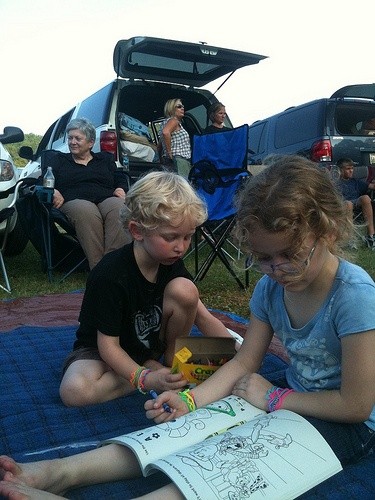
175 104 184 109
247 232 322 275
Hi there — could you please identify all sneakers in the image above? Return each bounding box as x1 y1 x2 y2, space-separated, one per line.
365 232 375 251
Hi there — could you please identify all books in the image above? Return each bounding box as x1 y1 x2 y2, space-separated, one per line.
96 394 343 500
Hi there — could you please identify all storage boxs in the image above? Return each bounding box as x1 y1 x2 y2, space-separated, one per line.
172 336 237 389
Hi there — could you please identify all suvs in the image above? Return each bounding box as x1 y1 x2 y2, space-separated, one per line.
247 82 375 184
18 35 270 192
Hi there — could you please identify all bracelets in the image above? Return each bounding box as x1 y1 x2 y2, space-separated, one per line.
265 386 292 411
129 366 152 397
178 389 197 413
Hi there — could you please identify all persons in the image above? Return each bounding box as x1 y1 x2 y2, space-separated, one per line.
0 157 375 500
158 98 192 180
60 173 232 406
35 119 132 273
203 102 234 135
335 159 375 251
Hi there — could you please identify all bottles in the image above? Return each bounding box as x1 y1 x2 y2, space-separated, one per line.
43 166 55 188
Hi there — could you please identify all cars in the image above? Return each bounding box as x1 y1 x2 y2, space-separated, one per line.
0 125 29 258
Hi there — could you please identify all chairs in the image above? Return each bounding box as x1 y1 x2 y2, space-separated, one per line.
188 124 254 289
16 150 117 285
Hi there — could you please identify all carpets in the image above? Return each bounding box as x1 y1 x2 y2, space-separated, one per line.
0 288 375 500
